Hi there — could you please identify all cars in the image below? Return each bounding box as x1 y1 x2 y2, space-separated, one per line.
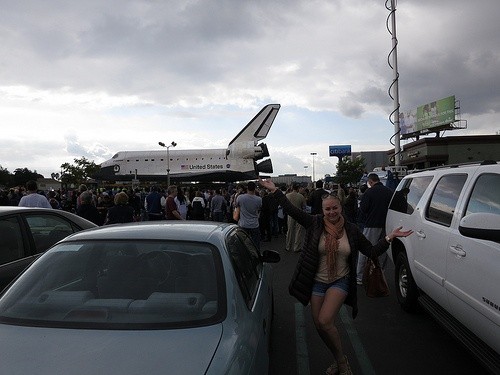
0 206 100 297
360 171 387 187
0 221 280 375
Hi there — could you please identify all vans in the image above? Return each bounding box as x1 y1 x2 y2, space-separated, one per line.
387 160 500 355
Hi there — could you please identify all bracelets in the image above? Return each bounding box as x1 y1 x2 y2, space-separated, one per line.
385 235 391 242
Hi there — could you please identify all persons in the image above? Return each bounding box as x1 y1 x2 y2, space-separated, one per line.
399 111 414 135
356 173 394 286
257 178 413 375
284 184 307 252
0 180 364 245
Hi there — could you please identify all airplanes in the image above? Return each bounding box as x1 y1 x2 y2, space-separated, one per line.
100 104 280 182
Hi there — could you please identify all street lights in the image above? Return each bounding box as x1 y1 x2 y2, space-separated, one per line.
304 166 309 175
159 142 177 195
310 152 317 182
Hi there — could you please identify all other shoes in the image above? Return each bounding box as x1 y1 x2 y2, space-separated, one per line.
263 239 270 242
325 355 352 375
356 277 363 284
